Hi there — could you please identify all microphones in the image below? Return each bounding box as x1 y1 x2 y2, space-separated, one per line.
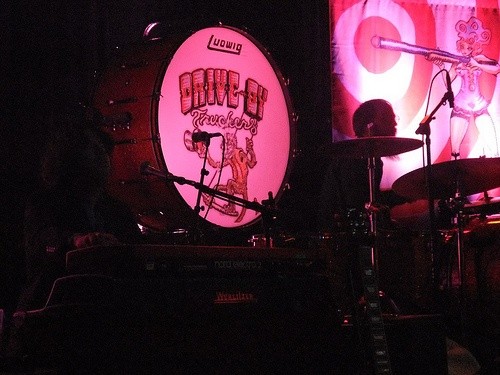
192 132 222 141
446 71 454 108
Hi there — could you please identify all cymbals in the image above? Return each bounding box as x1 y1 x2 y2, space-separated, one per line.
312 136 424 165
391 158 500 199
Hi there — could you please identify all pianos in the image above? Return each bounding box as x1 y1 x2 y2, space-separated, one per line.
64 241 321 375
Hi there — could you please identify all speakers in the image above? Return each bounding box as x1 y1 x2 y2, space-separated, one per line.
451 225 500 305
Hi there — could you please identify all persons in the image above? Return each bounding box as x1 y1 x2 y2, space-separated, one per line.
308 99 397 225
17 128 142 309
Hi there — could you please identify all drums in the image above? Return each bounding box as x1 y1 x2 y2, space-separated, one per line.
316 212 500 325
87 18 301 238
